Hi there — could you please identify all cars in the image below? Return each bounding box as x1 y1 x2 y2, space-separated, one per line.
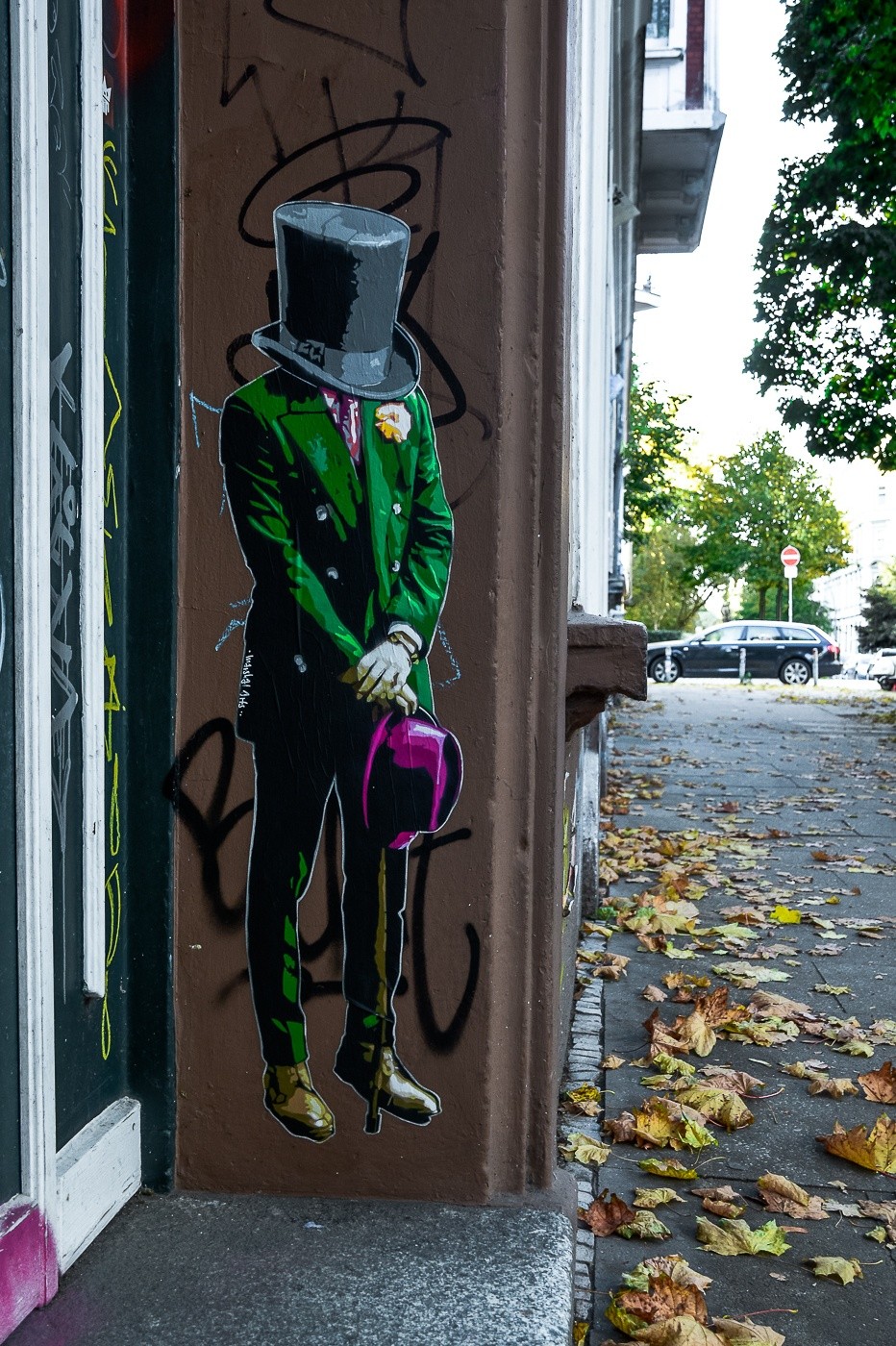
647 619 844 686
846 646 896 691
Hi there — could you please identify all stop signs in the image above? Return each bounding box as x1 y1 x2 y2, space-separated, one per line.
780 545 801 567
